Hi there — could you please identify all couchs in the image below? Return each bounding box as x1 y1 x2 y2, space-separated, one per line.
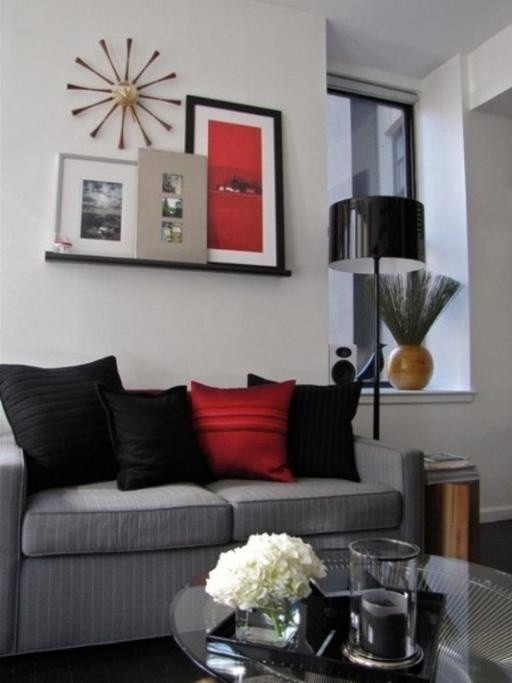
1 432 424 657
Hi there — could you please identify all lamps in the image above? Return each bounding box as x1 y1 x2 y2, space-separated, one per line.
328 195 426 441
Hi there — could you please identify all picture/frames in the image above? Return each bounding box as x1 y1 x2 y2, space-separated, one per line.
185 94 285 269
54 153 137 260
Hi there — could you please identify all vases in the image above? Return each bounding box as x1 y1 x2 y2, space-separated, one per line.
386 345 434 390
235 601 304 647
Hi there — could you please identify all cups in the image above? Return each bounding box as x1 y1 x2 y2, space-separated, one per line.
346 535 424 660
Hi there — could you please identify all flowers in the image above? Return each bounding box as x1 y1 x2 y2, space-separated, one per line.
204 532 328 637
